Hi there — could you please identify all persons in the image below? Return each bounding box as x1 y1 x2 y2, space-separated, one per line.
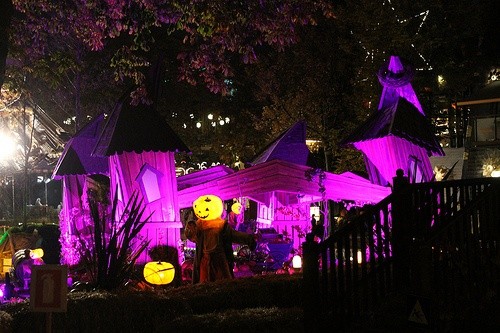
185 212 255 287
